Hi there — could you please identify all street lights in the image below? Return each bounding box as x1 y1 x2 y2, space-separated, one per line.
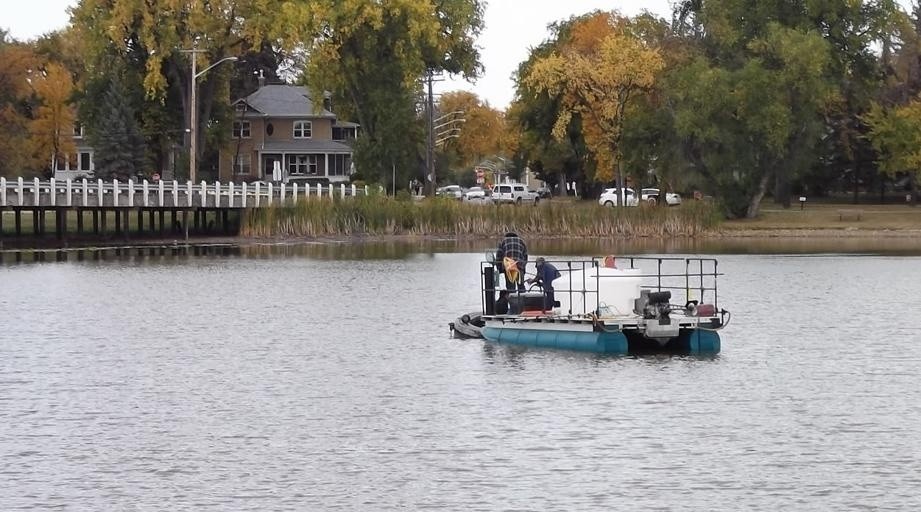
189 41 240 186
432 110 468 148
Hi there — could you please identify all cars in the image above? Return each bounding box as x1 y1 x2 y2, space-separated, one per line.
436 183 541 206
640 188 682 206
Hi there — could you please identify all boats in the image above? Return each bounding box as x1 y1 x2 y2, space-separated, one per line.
448 248 733 360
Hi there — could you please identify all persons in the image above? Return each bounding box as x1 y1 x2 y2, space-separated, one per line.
495 232 529 294
415 182 420 195
460 186 464 193
527 258 562 311
489 184 493 190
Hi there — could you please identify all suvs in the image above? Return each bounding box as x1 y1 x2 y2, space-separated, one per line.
598 187 637 209
